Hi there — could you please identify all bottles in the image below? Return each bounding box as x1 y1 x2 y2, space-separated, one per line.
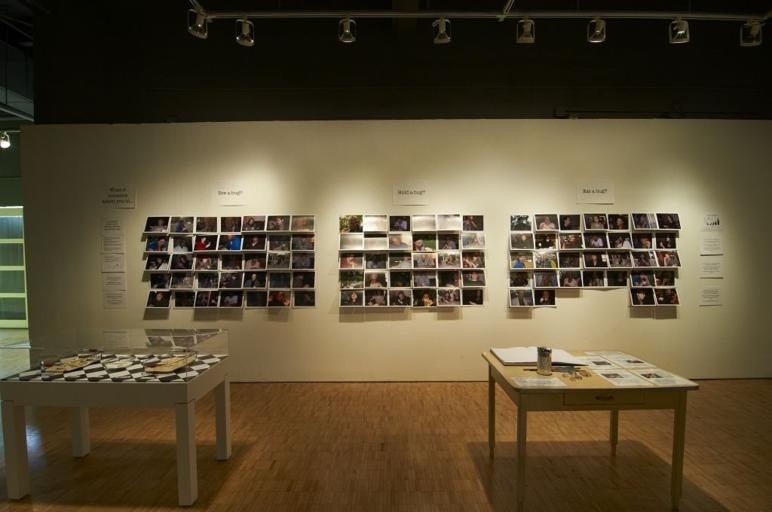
537 346 552 375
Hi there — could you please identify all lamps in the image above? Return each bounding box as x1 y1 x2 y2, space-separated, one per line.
183 1 772 47
0 131 11 148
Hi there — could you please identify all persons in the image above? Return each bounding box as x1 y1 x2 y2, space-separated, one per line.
147 217 314 307
341 215 483 304
511 216 677 305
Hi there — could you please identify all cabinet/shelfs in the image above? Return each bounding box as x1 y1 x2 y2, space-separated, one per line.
0 207 28 329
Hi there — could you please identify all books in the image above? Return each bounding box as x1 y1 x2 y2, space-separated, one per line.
490 345 587 366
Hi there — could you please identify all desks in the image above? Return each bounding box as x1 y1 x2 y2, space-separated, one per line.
1 346 233 507
483 351 700 512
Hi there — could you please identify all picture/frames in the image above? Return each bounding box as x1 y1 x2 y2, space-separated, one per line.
339 214 487 308
508 214 681 310
141 212 315 311
